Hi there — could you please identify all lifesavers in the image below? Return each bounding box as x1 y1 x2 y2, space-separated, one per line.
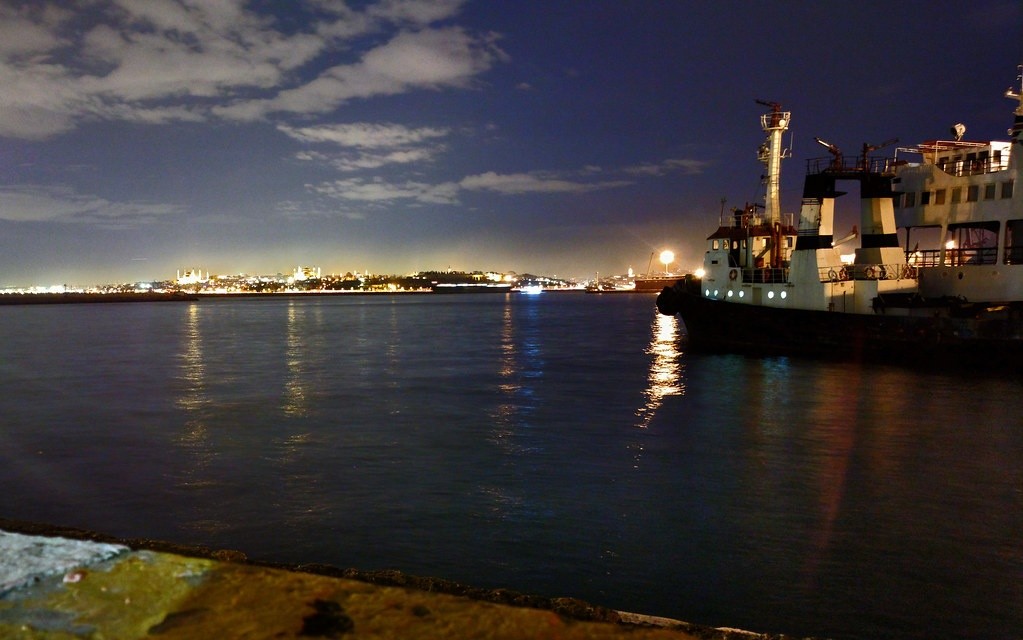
864 267 874 279
904 267 914 278
839 269 849 280
729 269 737 280
828 269 837 279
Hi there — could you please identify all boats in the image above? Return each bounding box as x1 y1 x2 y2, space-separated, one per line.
655 65 1023 362
433 282 514 294
